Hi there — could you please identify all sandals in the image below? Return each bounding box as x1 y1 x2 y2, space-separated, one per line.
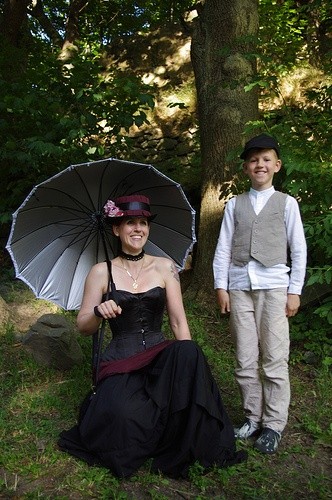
252 427 281 453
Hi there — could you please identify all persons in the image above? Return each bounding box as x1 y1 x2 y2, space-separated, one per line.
212 133 307 455
76 198 206 478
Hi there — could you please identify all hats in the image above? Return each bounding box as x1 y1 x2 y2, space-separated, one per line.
97 195 157 223
240 134 281 160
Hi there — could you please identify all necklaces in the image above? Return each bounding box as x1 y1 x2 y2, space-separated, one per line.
119 257 144 289
119 249 145 262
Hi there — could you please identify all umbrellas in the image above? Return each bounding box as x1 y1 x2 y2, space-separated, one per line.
5 157 198 319
